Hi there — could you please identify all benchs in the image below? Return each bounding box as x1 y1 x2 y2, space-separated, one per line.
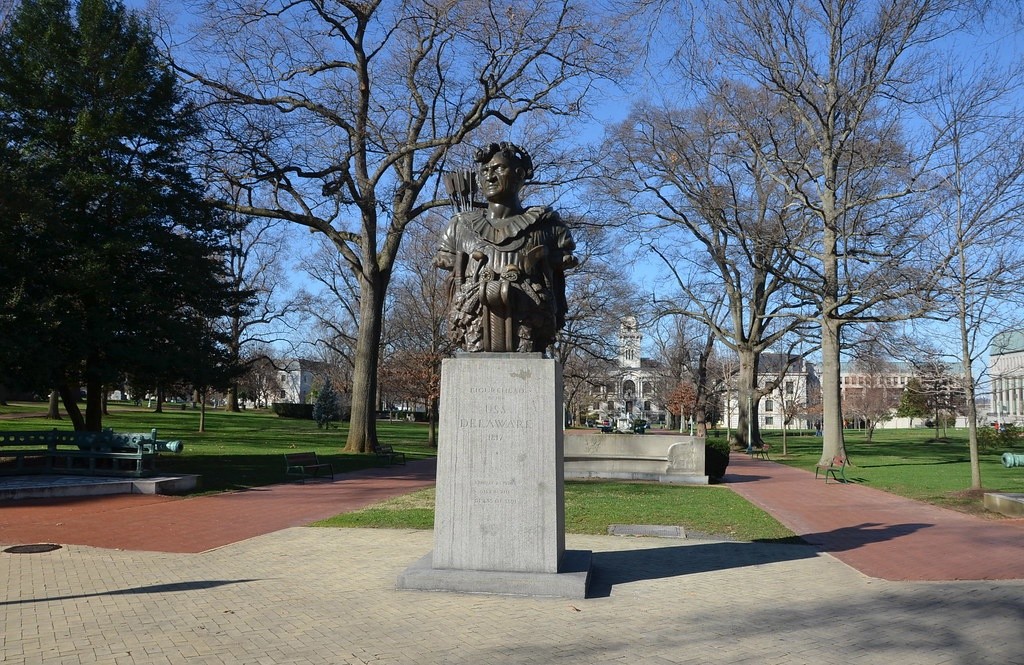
283 451 334 485
47 428 157 477
752 444 771 461
374 444 407 467
816 455 848 484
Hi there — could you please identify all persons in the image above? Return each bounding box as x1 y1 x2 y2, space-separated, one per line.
432 141 578 354
814 422 821 437
